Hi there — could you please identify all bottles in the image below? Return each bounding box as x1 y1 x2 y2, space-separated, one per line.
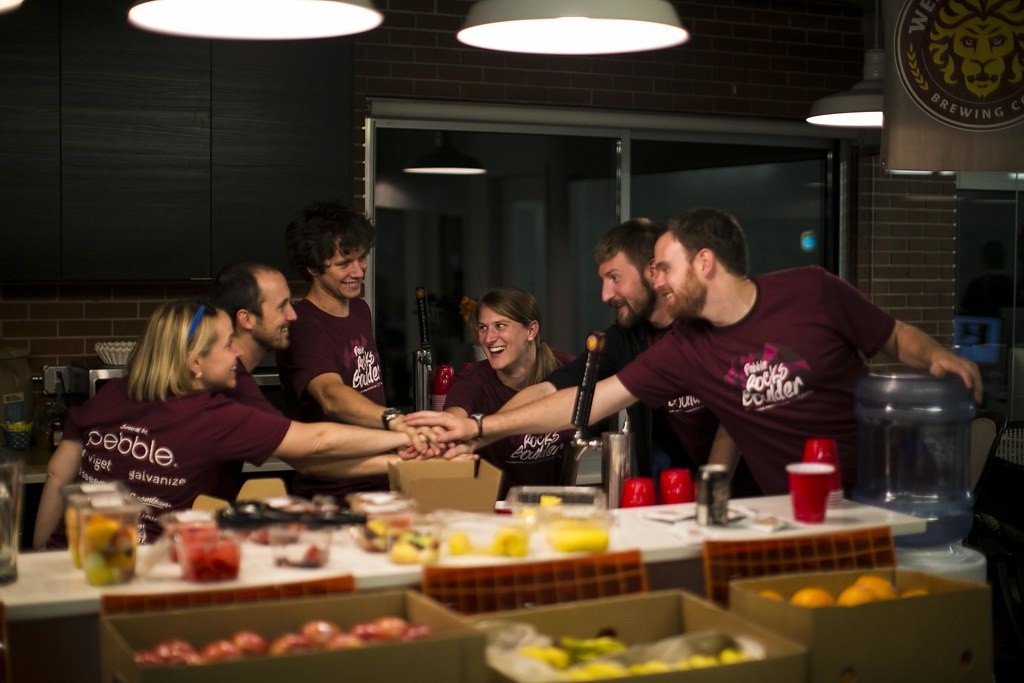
845 363 974 547
51 422 64 455
62 482 124 569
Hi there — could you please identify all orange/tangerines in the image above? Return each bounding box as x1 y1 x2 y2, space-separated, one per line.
758 576 929 607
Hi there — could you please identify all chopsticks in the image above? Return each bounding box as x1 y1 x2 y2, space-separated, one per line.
93 341 137 366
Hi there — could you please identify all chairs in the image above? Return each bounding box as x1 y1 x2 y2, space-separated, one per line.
970 408 1024 662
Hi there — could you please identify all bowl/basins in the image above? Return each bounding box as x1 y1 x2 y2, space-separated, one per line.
541 503 614 551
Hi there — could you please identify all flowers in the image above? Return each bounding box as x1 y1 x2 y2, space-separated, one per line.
459 296 480 346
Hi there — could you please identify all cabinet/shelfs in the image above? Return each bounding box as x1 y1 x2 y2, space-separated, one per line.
1 0 356 282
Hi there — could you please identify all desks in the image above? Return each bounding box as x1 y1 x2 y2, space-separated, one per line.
0 494 928 621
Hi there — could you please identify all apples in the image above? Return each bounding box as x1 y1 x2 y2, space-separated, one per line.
136 617 433 664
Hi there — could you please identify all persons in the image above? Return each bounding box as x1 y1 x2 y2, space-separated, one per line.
33 207 984 551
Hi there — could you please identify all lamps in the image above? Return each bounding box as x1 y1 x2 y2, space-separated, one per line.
457 0 690 55
805 1 885 128
401 130 487 175
127 0 384 41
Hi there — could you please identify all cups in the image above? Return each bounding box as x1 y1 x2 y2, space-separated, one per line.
0 457 24 586
786 463 835 523
161 511 216 563
361 510 416 553
386 523 441 565
662 469 692 504
697 464 731 527
175 529 240 582
804 439 844 508
433 366 454 411
620 478 657 509
461 362 472 375
78 506 144 586
252 499 301 545
269 523 332 567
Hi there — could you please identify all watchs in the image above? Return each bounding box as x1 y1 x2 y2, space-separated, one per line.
382 408 401 430
470 412 484 438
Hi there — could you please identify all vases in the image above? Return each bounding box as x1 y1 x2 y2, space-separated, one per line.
472 345 487 361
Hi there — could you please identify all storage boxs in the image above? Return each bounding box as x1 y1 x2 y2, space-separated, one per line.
101 589 487 683
729 566 995 683
470 587 811 682
389 458 502 512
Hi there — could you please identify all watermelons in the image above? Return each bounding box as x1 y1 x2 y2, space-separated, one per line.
169 526 238 581
